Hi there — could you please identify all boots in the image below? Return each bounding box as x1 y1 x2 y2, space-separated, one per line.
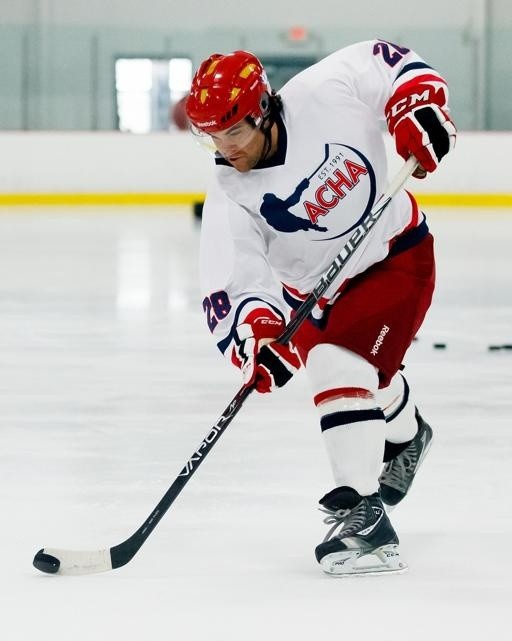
379 406 435 504
314 487 398 563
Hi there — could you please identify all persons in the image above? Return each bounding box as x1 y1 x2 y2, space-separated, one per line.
182 36 457 573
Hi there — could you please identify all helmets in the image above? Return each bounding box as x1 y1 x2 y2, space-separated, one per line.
187 51 273 162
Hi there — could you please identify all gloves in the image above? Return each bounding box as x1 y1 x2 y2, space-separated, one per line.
381 77 458 182
230 303 303 396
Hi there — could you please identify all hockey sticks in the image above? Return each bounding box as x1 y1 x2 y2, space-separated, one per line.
33 154 420 575
